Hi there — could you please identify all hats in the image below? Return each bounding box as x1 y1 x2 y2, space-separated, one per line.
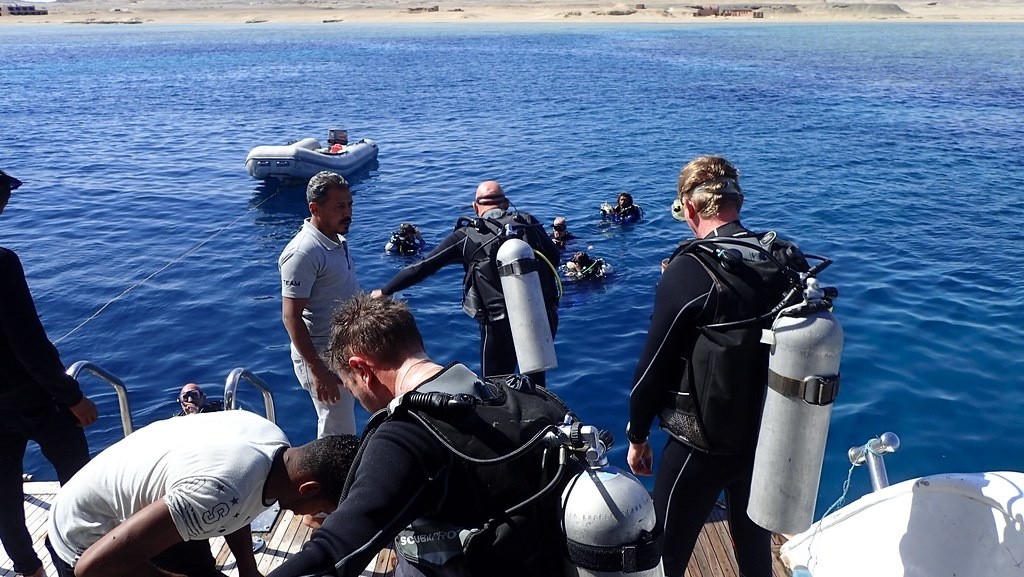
0 170 22 190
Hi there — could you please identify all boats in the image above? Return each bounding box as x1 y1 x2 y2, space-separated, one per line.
244 128 378 185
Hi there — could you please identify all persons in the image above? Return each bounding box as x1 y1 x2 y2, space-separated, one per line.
0 170 99 577
44 408 360 577
626 156 818 577
266 171 643 577
177 384 224 417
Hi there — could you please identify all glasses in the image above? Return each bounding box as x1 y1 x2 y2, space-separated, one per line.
178 390 201 403
